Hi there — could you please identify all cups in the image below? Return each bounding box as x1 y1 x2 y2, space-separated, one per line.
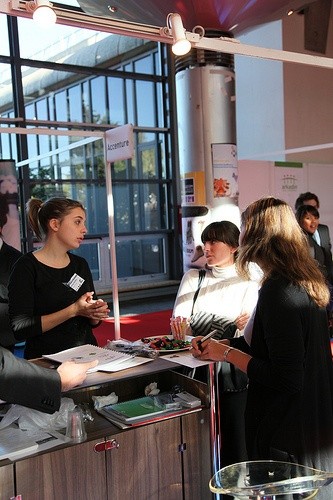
64 410 87 443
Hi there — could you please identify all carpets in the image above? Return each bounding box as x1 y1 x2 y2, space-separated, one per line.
92 310 172 347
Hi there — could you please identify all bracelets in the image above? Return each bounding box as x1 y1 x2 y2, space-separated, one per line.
223 347 236 363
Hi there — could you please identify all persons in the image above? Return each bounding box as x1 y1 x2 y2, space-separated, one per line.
171 221 265 500
0 193 24 355
188 192 333 500
0 346 98 414
7 197 110 360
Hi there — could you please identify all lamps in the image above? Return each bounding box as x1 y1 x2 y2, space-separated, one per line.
166 13 191 56
26 0 57 24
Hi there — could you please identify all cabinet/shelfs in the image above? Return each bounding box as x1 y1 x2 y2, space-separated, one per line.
0 345 216 500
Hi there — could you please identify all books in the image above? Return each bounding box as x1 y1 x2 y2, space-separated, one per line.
0 423 38 461
96 392 202 429
42 343 136 373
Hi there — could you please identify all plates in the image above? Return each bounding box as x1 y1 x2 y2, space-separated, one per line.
132 335 196 352
